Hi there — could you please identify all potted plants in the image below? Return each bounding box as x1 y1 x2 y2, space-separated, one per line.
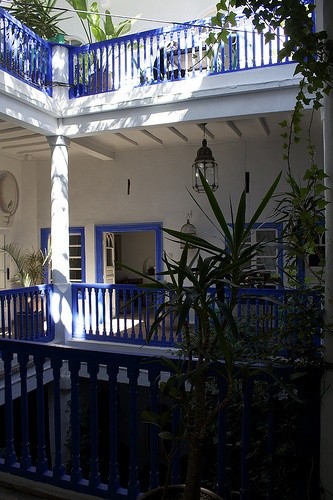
64 0 133 96
115 169 305 500
0 242 53 347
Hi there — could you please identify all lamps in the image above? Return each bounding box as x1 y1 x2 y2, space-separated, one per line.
190 123 218 194
178 211 197 251
